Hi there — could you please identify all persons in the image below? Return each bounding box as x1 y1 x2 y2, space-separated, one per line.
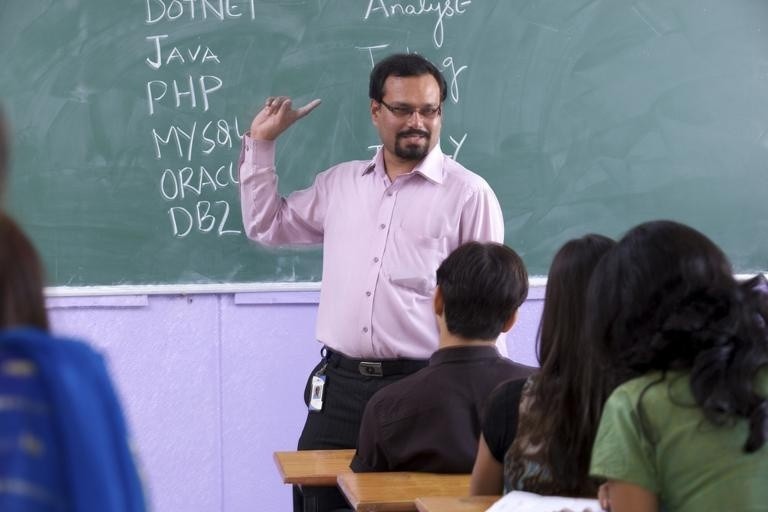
312 386 321 399
584 219 768 511
239 53 505 512
465 232 618 498
350 239 540 476
2 113 149 512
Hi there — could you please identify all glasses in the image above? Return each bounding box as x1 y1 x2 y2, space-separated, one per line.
380 99 441 117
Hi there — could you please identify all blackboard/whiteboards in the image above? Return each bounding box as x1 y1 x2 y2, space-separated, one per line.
0 0 768 296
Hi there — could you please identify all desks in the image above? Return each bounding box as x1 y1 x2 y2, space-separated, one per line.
272 446 502 512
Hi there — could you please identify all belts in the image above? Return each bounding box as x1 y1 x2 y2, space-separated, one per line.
325 347 430 377
